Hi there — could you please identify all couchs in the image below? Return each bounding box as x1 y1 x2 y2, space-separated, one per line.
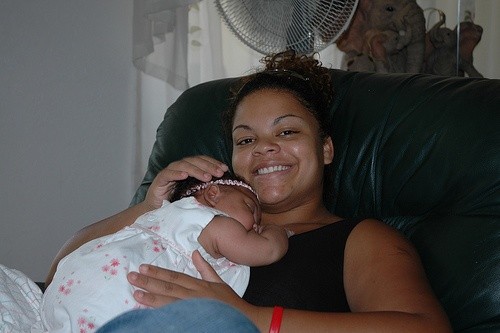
125 69 500 333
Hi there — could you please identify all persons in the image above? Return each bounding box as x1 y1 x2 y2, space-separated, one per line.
41 171 291 333
45 49 453 333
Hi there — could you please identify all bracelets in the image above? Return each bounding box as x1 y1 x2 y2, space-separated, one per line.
267 305 285 333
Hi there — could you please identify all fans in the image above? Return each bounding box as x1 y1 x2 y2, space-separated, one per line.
215 0 362 64
